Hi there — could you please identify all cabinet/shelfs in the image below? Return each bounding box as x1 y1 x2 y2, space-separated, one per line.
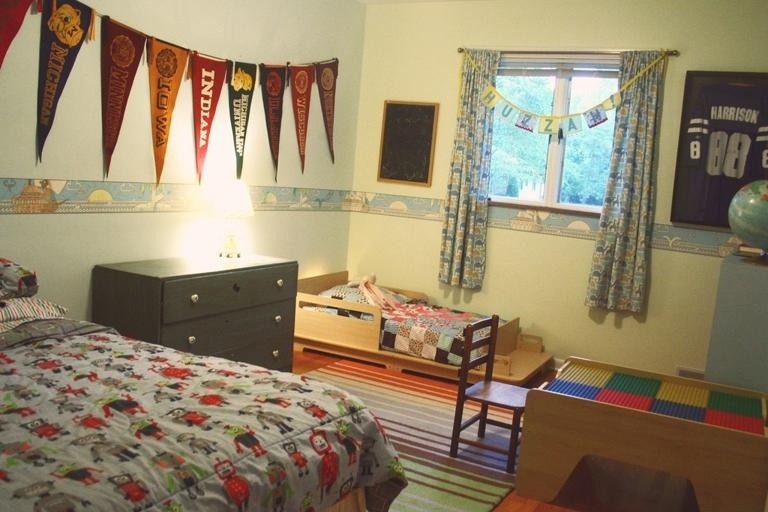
93 253 302 376
700 248 768 396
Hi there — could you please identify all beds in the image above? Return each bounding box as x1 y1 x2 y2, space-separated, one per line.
294 266 556 387
1 291 416 511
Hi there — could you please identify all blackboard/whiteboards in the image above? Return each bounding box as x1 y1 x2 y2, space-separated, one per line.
377 100 438 187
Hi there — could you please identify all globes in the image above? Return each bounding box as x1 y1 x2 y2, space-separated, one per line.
728 180 768 251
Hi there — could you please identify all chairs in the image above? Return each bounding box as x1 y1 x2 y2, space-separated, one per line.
450 313 537 476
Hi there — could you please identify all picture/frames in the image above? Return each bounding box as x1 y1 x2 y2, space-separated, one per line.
373 97 443 190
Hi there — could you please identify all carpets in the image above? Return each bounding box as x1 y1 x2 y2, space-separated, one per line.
298 357 523 511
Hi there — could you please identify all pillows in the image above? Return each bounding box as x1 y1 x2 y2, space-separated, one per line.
0 255 40 301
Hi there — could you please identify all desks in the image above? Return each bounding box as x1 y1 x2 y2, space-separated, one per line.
514 351 768 511
